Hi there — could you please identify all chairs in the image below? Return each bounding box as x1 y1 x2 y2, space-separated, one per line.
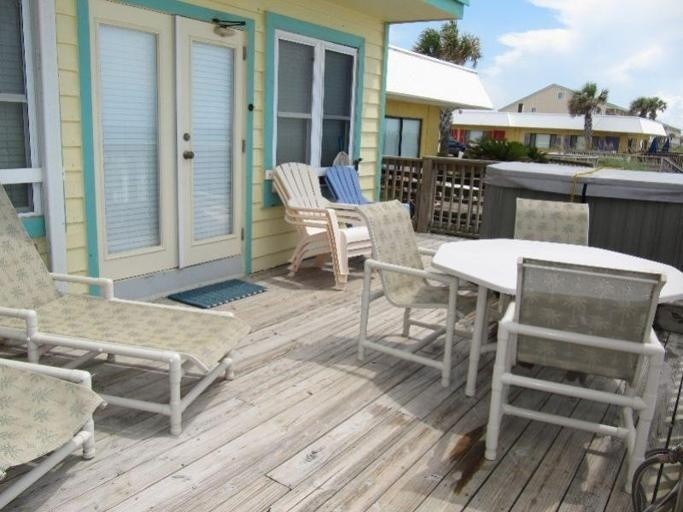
273 163 410 292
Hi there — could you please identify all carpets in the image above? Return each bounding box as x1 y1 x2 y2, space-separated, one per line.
168 279 267 307
